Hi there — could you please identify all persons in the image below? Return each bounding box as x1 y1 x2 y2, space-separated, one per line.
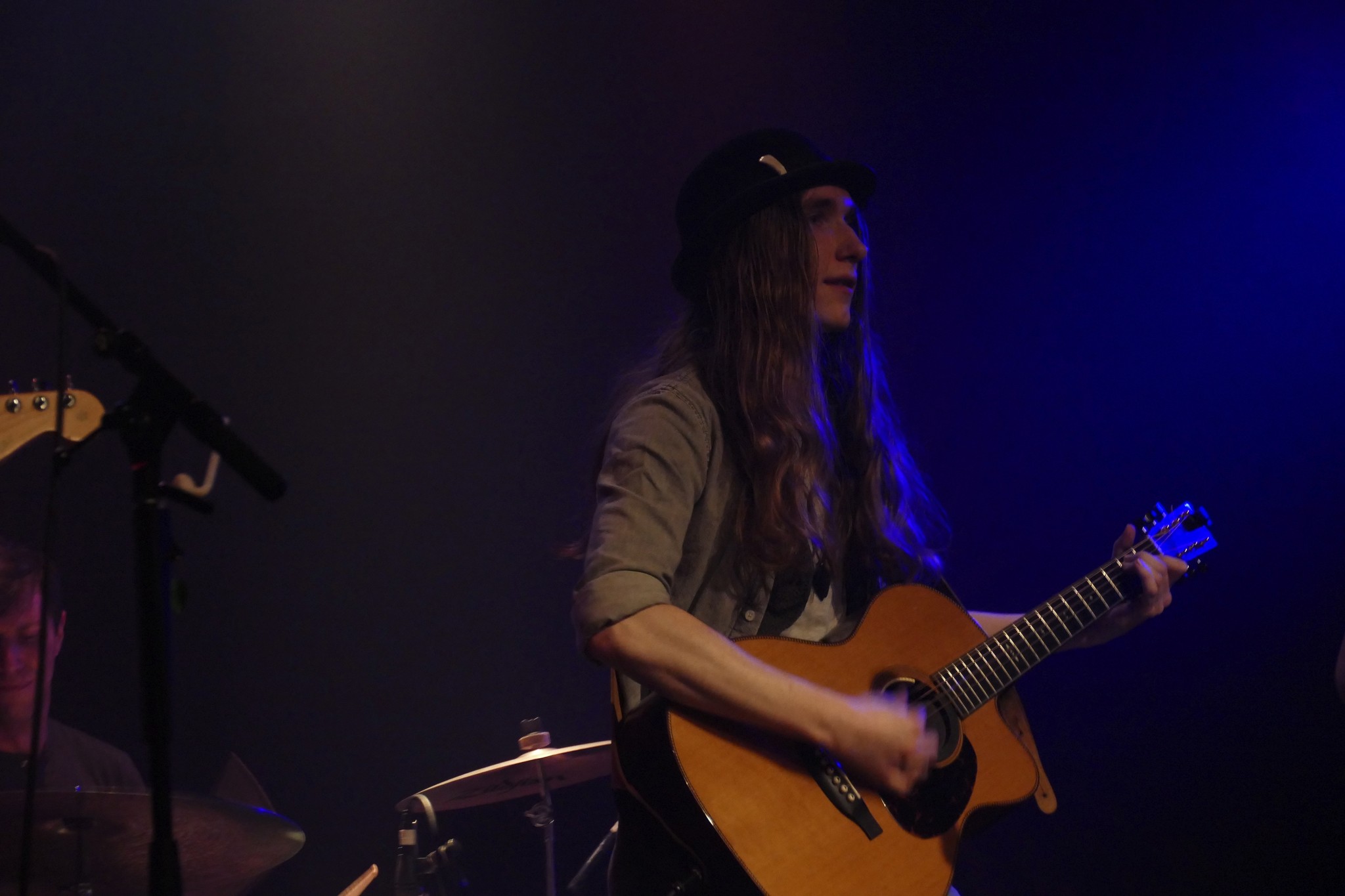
0 535 144 796
577 134 1189 896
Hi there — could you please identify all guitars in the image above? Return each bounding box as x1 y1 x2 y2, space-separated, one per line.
1 371 109 467
664 496 1223 896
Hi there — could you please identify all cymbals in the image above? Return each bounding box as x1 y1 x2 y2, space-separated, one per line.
395 736 615 819
0 782 307 895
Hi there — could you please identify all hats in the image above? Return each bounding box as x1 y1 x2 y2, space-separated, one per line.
676 128 869 296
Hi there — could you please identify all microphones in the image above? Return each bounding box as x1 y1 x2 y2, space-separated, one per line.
393 810 423 896
